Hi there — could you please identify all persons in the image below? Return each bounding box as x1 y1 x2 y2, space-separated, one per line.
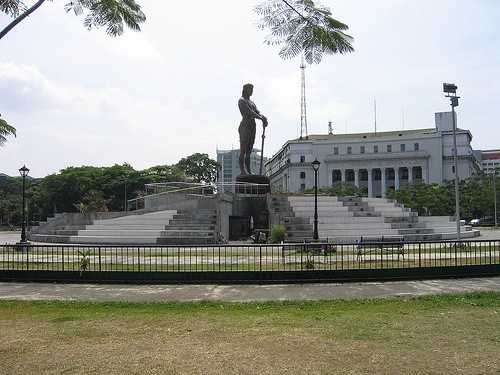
237 83 268 175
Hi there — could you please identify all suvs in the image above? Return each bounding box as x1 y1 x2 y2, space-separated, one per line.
468 219 481 227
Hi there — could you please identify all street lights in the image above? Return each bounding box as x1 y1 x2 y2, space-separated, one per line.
311 157 321 241
443 83 460 248
16 164 31 245
